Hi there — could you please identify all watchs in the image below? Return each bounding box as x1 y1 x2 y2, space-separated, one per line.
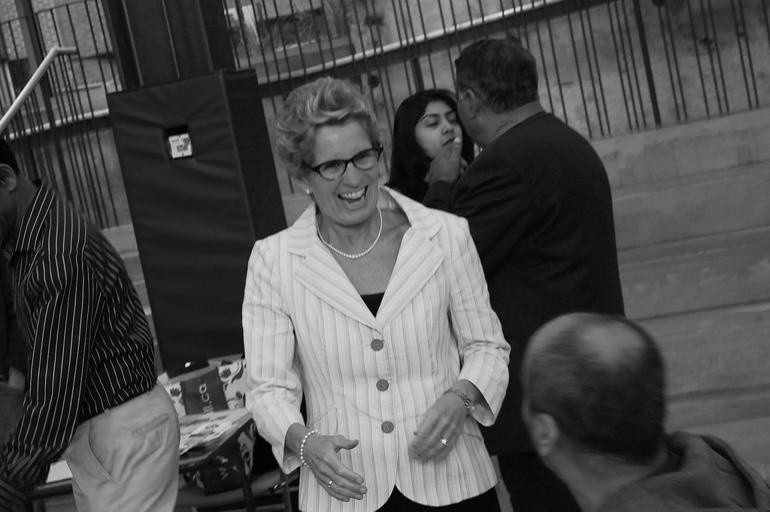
441 385 478 417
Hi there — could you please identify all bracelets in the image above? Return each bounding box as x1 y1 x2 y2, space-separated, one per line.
299 429 322 469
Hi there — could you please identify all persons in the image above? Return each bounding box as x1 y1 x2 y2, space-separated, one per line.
0 130 182 510
518 310 770 512
421 37 627 511
242 74 515 512
376 86 474 201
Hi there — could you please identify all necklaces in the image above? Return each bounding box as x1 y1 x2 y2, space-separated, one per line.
314 201 384 260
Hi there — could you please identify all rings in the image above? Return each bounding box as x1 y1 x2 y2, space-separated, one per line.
437 435 452 450
327 479 334 490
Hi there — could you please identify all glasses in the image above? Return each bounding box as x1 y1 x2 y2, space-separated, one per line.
308 143 383 181
